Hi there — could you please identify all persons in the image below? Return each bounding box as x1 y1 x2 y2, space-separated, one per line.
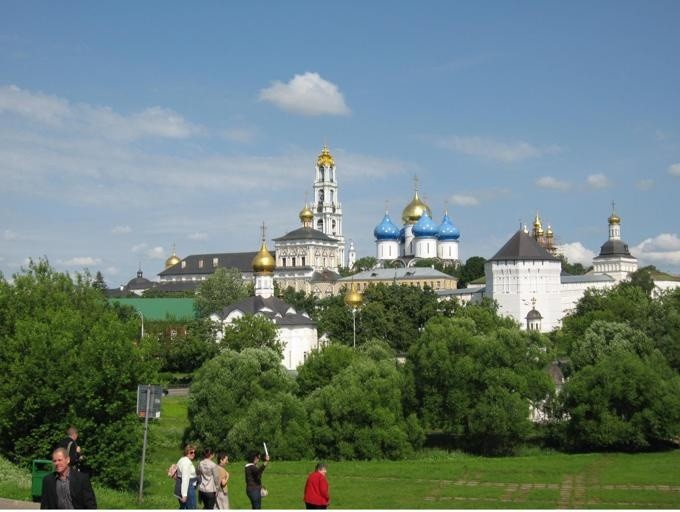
213 450 230 509
40 446 98 509
60 426 83 471
174 443 198 509
196 446 219 509
304 462 331 510
245 449 270 509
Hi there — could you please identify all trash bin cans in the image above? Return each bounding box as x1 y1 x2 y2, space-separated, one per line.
31 460 56 502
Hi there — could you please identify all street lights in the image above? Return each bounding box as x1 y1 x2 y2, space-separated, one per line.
137 310 146 342
352 308 358 349
